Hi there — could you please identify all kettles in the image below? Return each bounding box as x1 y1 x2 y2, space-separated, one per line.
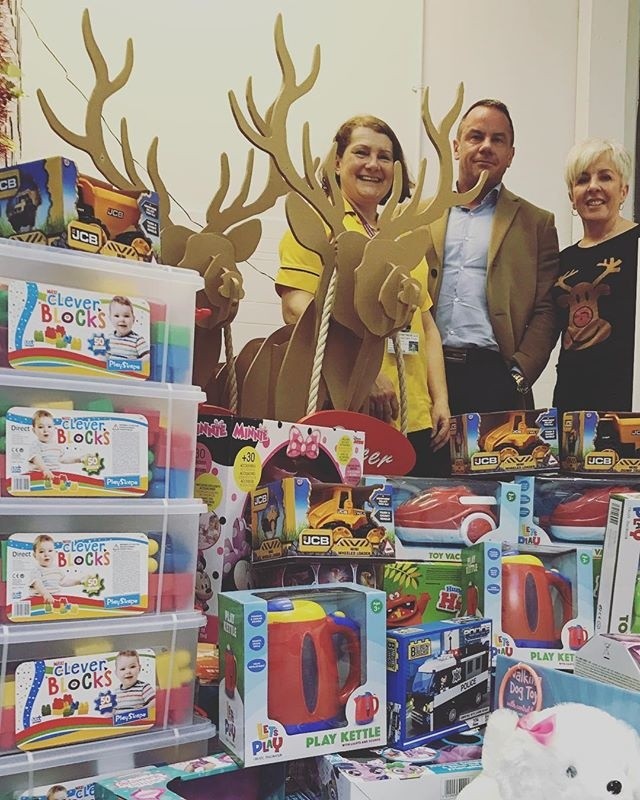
224 644 237 699
467 581 479 617
267 597 361 735
568 625 588 651
354 692 379 725
502 549 572 649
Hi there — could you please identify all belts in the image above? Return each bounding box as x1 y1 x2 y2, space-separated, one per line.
442 344 503 364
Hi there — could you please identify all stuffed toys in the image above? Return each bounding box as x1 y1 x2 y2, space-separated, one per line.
455 702 640 800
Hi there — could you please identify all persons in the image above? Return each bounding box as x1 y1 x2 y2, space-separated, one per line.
29 535 85 603
48 785 68 800
401 99 562 416
275 114 452 480
113 649 156 714
108 295 150 361
28 410 85 478
548 137 640 413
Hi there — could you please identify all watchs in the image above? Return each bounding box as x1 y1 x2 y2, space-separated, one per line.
511 370 529 394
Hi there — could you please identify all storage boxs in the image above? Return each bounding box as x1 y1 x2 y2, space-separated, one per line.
0 156 640 798
